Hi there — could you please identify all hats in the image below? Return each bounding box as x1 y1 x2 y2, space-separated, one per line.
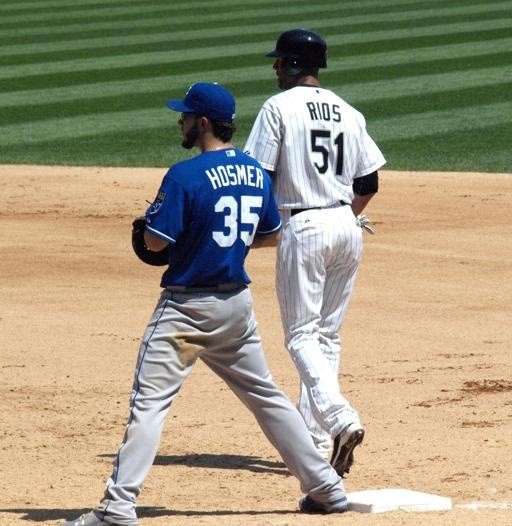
166 81 236 120
264 28 327 58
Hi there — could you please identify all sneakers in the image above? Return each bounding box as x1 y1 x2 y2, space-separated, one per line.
298 417 365 513
59 504 140 526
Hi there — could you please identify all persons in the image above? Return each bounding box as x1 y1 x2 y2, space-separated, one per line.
74 83 348 526
243 29 386 480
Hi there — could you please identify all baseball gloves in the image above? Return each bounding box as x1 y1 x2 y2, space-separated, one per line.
132 216 168 266
